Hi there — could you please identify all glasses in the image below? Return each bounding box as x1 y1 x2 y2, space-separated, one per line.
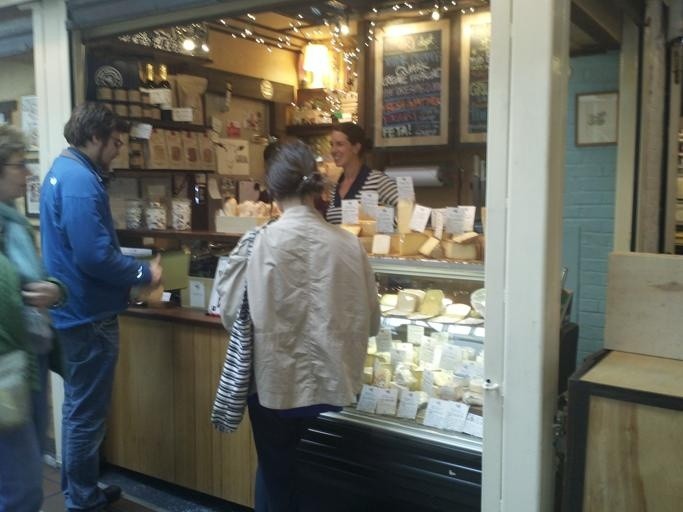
110 136 124 149
0 159 28 169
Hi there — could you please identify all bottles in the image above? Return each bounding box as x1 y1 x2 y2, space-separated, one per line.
144 63 172 120
125 198 167 230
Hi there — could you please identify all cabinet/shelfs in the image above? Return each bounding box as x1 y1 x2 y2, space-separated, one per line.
101 73 224 176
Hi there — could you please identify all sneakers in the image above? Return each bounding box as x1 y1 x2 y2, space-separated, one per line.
66 483 122 512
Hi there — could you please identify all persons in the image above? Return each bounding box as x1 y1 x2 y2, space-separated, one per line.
325 122 398 226
0 121 68 512
38 100 164 512
216 139 382 511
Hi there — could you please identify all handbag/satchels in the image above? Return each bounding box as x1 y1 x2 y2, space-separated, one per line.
208 226 257 438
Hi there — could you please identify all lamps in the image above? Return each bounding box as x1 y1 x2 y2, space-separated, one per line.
303 46 331 89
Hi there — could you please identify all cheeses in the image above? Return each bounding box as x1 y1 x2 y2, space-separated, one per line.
339 218 486 413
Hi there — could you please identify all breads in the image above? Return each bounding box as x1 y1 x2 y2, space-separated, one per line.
224 197 279 219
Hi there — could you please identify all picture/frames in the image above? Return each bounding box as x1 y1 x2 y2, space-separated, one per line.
24 157 41 218
574 90 618 146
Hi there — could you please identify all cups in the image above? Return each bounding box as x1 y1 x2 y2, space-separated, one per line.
171 199 193 230
